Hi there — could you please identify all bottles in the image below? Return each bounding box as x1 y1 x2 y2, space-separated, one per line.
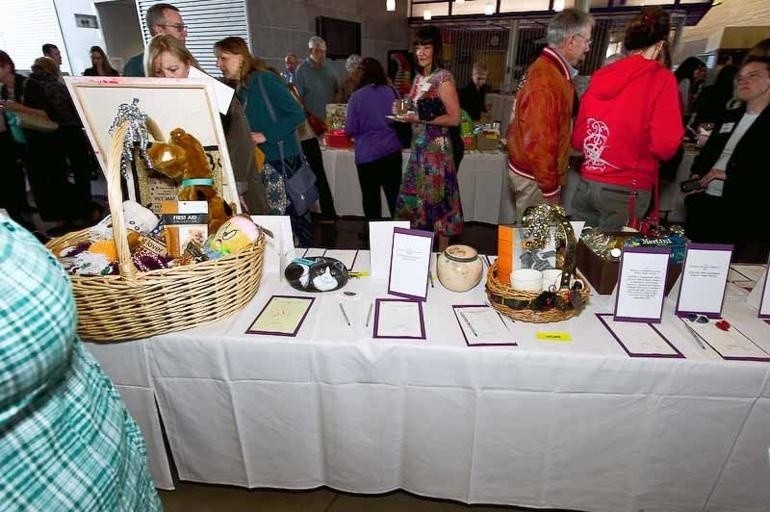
462 117 501 136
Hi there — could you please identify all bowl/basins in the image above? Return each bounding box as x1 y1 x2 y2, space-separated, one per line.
323 129 353 147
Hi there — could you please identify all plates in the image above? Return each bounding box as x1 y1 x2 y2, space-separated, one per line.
385 115 413 123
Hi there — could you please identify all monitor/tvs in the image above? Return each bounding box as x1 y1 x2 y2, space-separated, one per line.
316 16 362 59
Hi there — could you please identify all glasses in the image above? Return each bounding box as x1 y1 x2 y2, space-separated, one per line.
158 24 189 33
575 33 595 46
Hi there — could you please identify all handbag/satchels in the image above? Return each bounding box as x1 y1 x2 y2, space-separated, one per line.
286 161 320 217
416 96 446 121
391 120 410 149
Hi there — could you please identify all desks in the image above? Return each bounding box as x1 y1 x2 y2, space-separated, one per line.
300 141 504 225
82 245 768 512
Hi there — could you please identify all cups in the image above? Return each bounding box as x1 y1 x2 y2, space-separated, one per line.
395 99 408 115
510 268 584 295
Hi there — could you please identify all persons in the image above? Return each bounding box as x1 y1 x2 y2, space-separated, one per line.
117 4 189 77
567 8 687 240
342 56 403 238
0 209 165 511
673 37 769 142
504 7 596 228
209 35 337 248
143 31 273 216
392 25 465 251
458 58 492 123
342 53 364 103
294 36 343 129
0 44 119 240
680 54 770 267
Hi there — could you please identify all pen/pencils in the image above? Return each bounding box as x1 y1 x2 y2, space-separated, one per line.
366 304 373 327
460 311 478 337
339 303 350 327
686 325 706 350
482 254 491 268
430 272 434 288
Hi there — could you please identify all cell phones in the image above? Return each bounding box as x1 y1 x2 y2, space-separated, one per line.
680 177 709 194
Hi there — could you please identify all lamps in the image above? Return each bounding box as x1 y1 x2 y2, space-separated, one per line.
376 0 574 23
